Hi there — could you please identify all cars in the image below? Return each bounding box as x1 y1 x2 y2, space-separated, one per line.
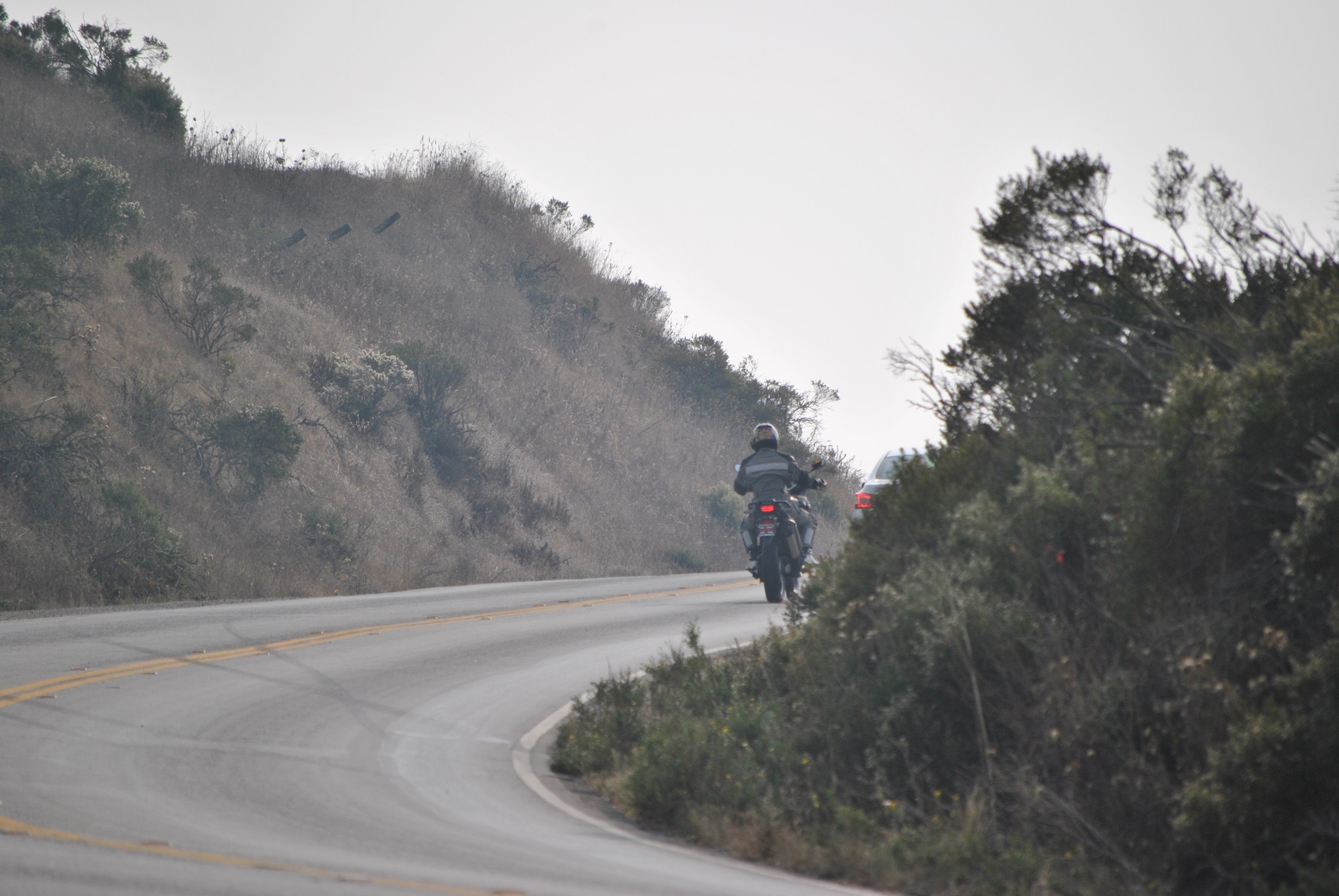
848 448 939 535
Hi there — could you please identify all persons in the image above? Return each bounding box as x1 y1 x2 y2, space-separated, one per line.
734 423 824 569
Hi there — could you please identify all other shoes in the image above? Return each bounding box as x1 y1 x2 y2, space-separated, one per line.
745 561 759 571
803 552 819 566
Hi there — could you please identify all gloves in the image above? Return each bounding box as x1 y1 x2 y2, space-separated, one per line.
812 477 824 490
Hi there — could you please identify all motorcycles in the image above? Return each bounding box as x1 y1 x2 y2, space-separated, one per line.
734 457 826 603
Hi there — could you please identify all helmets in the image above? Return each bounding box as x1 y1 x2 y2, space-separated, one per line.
753 424 780 450
